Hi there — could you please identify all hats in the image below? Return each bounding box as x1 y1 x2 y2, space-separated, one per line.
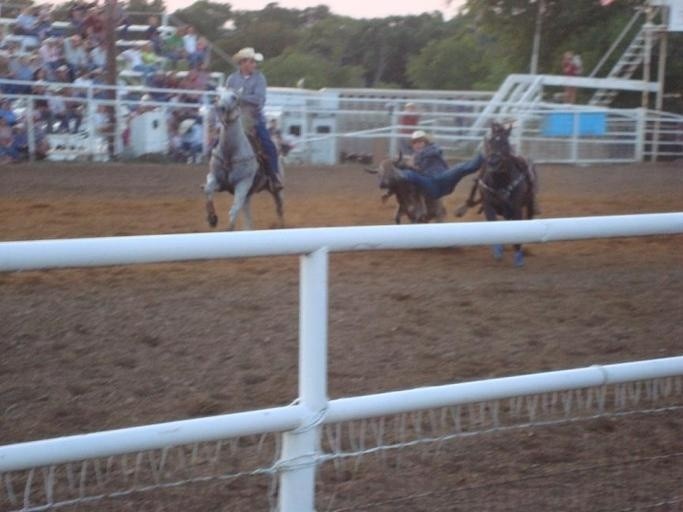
407 129 434 147
28 32 97 88
177 116 196 135
231 46 265 65
403 101 417 110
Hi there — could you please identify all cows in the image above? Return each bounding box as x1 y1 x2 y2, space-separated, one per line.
361 149 447 225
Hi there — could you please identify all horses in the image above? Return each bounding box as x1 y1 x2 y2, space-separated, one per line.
476 120 530 271
201 84 288 231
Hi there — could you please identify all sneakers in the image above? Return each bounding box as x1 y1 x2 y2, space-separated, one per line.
264 175 284 192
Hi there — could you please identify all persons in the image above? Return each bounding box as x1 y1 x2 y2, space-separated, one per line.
209 46 284 194
397 130 486 202
560 46 583 103
1 1 287 163
399 104 420 163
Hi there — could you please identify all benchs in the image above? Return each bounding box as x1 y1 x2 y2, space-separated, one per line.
1 12 226 154
